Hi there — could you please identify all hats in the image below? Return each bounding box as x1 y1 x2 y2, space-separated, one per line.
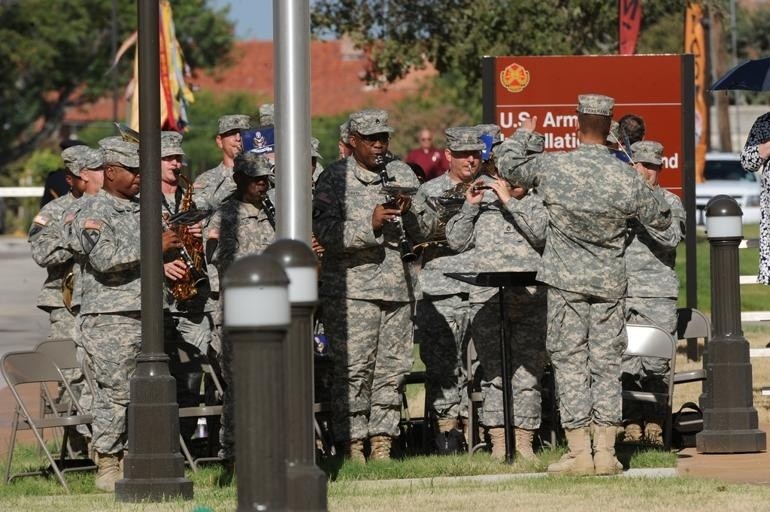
217 115 249 135
350 109 394 136
310 136 323 160
98 136 122 151
575 93 616 117
104 142 140 169
259 104 275 126
627 139 664 167
160 131 184 160
78 149 104 174
524 133 545 153
444 127 483 152
607 119 619 145
232 152 275 178
340 121 348 144
61 144 90 177
477 123 503 146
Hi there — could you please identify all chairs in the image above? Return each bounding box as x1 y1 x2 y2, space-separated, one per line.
0 350 100 502
398 370 437 457
35 338 98 467
177 362 225 477
464 338 491 457
619 325 673 450
676 309 710 382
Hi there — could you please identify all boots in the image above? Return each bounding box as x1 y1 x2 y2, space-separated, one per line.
67 429 90 463
593 426 623 475
547 425 594 475
513 427 544 470
623 423 643 445
369 435 392 466
487 427 506 465
644 422 665 446
96 450 129 493
434 417 465 458
339 439 365 469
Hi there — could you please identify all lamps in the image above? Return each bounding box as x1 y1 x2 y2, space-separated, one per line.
698 192 738 411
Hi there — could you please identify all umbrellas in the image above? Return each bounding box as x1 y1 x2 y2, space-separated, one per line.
706 57 770 93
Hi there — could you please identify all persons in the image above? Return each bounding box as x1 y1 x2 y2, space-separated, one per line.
740 112 770 288
239 124 277 164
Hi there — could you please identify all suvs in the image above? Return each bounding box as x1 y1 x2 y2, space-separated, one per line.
697 152 764 225
